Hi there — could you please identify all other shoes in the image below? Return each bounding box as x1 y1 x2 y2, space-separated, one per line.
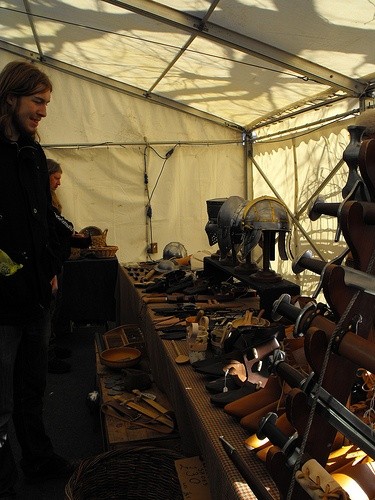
24 453 75 483
191 326 375 500
48 354 74 374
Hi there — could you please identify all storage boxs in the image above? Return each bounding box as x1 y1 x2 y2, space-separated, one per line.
63 445 190 500
71 229 118 258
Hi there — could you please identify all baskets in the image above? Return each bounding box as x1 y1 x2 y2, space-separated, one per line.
90 229 108 246
64 445 191 500
90 246 118 258
70 247 80 259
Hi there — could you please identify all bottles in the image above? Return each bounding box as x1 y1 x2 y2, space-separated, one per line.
187 331 208 364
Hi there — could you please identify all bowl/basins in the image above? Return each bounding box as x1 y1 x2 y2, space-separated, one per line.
100 347 142 369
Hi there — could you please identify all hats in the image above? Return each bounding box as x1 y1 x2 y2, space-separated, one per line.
154 260 179 274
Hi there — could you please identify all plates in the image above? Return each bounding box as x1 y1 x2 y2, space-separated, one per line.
232 316 265 330
265 319 270 328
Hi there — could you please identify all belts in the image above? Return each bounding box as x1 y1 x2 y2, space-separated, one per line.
101 399 173 433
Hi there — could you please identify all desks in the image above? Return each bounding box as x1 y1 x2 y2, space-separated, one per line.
115 261 280 500
63 258 118 324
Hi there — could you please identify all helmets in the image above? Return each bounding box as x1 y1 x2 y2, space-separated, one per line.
216 196 244 229
230 200 252 228
239 196 292 232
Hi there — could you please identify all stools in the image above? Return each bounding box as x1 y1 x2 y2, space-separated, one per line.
93 330 180 445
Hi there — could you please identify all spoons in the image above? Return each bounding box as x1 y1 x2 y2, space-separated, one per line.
247 312 252 326
242 310 249 326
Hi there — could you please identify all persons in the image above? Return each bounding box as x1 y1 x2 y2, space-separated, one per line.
0 61 78 480
45 159 70 374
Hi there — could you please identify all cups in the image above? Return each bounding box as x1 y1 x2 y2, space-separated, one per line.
186 325 205 333
185 316 197 333
